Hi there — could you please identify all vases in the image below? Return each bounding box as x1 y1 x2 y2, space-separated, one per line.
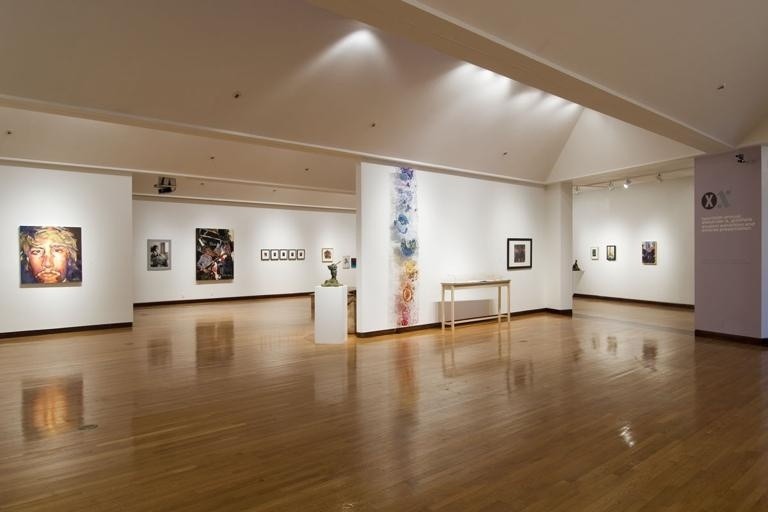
147 239 171 271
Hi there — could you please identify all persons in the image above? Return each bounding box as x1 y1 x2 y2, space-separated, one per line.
642 242 655 263
19 227 79 283
149 245 169 266
197 241 233 280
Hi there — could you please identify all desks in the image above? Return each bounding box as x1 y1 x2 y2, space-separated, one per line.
439 278 511 331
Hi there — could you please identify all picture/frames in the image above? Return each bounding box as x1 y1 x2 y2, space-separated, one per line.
321 248 334 262
507 238 532 269
260 249 305 261
606 245 616 260
